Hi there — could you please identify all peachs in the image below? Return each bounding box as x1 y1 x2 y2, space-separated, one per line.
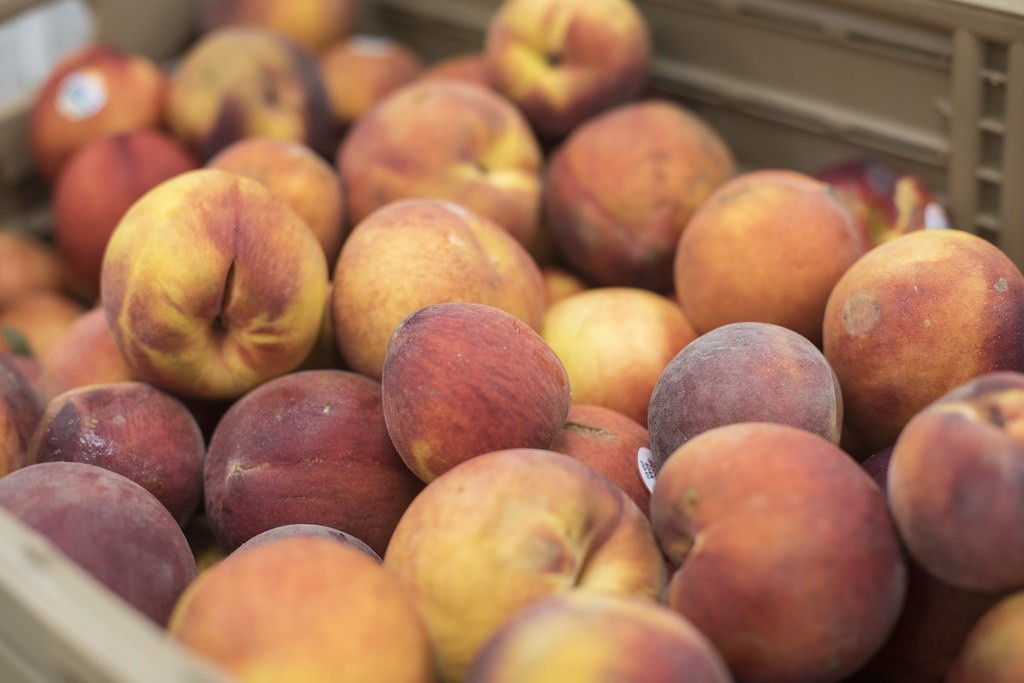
0 0 1024 683
